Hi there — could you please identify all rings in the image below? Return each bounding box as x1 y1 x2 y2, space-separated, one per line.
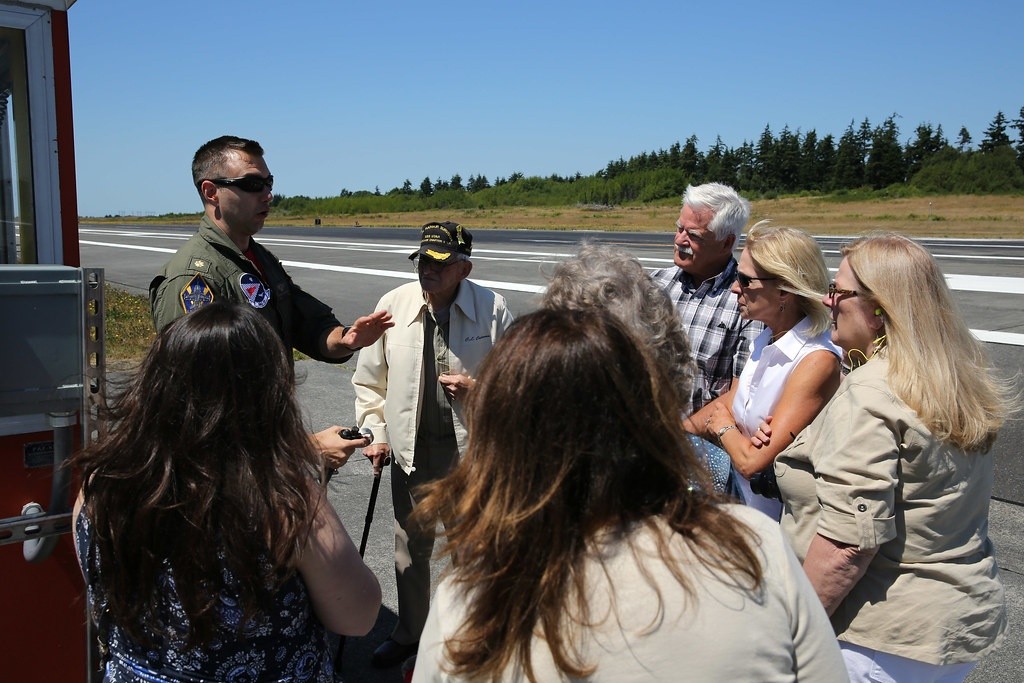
704 419 710 426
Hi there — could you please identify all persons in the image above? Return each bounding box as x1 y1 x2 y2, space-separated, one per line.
678 218 852 523
772 228 1022 683
409 237 852 683
148 135 395 485
647 182 767 437
351 221 513 664
52 303 382 683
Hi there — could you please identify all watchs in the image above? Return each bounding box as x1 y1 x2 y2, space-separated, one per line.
717 426 737 442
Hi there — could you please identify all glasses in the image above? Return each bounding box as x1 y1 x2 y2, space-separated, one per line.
411 257 466 273
197 172 274 193
734 265 777 287
828 283 863 298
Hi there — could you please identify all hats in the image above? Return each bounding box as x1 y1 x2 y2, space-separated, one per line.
408 220 474 261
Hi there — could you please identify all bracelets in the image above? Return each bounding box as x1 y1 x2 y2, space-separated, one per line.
342 324 362 351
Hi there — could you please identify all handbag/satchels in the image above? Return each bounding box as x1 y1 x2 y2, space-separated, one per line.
679 429 732 497
